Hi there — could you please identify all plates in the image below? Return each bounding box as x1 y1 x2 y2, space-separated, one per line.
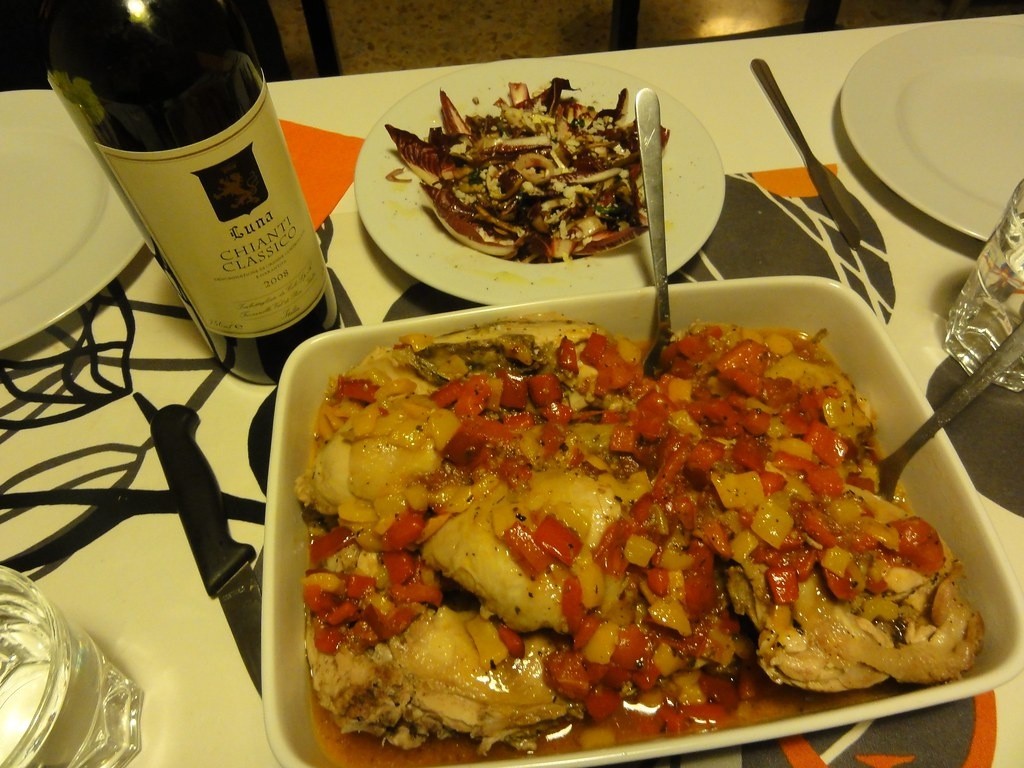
0 89 146 352
837 15 1024 242
353 57 726 306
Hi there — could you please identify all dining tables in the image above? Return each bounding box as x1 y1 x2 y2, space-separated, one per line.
0 15 1024 768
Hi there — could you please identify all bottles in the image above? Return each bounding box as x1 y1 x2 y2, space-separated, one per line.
40 0 342 385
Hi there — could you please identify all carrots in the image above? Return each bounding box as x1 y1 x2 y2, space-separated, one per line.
301 333 946 735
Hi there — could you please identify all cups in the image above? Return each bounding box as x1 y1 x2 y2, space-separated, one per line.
0 564 144 768
943 179 1024 394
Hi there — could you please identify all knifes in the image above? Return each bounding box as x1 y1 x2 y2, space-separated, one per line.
150 403 262 699
749 58 861 251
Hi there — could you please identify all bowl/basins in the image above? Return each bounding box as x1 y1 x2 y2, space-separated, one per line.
258 274 1024 768
